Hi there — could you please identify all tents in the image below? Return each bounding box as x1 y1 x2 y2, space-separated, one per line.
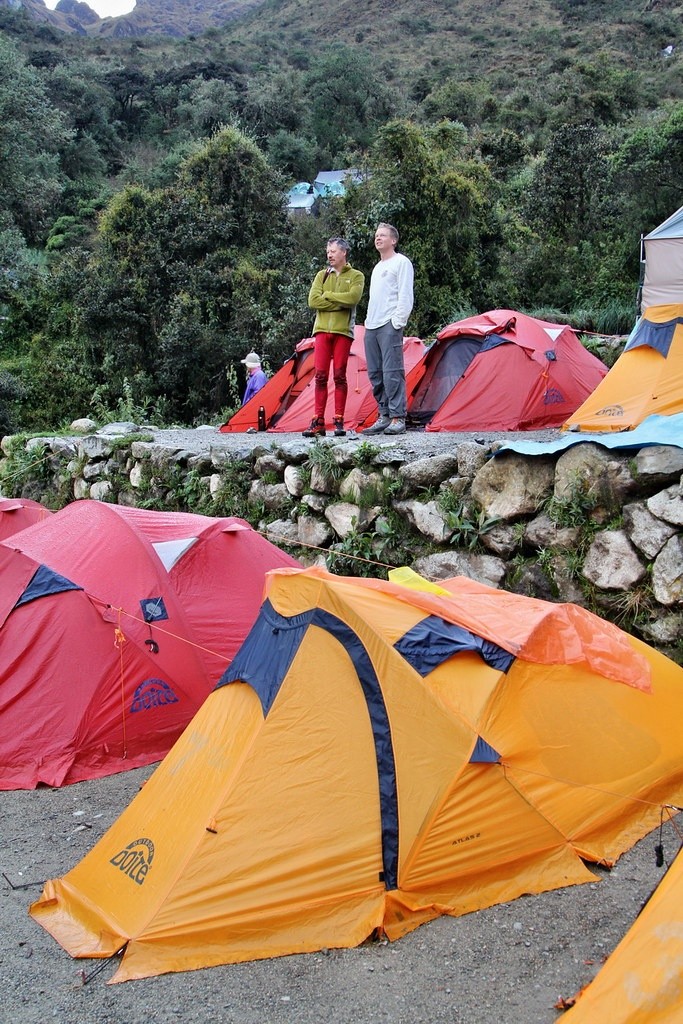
0 499 683 1024
218 308 682 431
637 202 683 303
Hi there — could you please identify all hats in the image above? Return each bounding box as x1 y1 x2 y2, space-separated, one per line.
241 353 260 368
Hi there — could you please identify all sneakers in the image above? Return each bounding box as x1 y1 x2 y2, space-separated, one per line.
383 417 406 434
302 417 326 437
362 416 391 435
333 416 346 436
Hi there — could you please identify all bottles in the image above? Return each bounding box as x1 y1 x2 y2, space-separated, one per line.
258 405 266 431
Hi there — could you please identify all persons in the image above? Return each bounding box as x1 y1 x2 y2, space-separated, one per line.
365 223 415 434
239 353 267 403
302 239 365 437
